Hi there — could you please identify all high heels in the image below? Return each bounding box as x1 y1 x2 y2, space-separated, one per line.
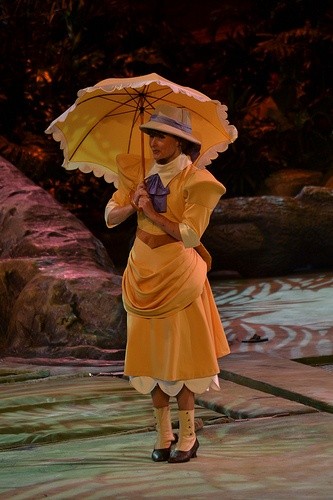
151 433 178 462
167 437 200 463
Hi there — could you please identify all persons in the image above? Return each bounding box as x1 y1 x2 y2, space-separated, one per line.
105 104 231 463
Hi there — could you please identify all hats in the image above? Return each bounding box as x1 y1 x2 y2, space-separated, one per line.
138 104 201 152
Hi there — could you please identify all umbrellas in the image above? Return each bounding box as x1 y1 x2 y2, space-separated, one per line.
45 73 238 212
